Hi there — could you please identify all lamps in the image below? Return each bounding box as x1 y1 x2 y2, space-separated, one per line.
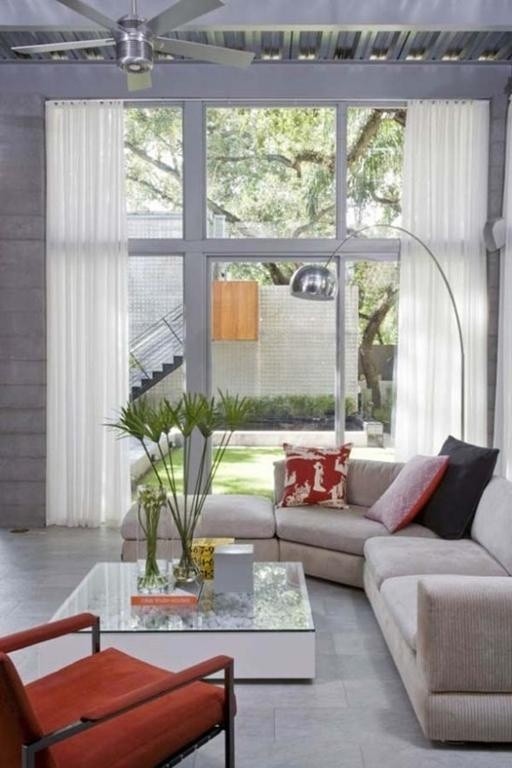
290 223 466 441
483 217 505 252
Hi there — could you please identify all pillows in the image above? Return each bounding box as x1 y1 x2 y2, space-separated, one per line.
364 453 449 533
413 435 499 539
273 441 353 509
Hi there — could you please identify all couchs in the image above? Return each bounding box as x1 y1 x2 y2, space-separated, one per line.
121 461 512 743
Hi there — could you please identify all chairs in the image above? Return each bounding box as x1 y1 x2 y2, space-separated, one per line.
0 613 237 768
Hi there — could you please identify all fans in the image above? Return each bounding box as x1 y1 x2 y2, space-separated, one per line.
12 0 256 93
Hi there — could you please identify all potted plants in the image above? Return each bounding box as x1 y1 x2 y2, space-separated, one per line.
103 388 254 582
136 484 168 594
